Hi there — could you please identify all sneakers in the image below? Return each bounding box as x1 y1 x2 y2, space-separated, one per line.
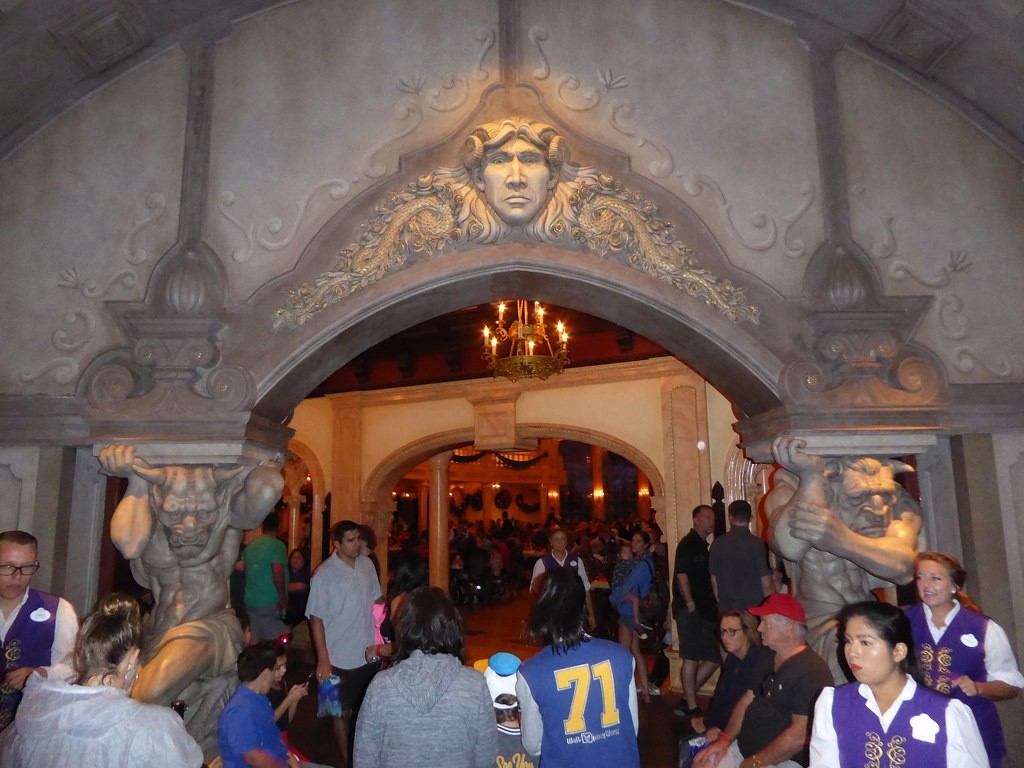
636 685 661 695
640 620 654 630
634 628 649 639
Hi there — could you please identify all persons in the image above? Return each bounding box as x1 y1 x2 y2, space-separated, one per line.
0 501 1024 768
463 115 572 223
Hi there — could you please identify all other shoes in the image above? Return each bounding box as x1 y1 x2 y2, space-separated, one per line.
687 707 705 735
636 696 651 703
672 699 690 717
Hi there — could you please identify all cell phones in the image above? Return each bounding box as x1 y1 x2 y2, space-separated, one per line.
303 672 313 687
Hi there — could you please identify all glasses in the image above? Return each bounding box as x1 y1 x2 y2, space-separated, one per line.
720 628 746 636
0 560 40 576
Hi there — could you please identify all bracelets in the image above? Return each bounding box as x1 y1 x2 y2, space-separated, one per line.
719 730 734 744
375 644 384 657
752 755 761 768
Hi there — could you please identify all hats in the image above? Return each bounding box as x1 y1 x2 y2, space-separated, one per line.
748 593 806 622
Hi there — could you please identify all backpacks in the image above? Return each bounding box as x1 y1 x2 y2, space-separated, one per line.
629 560 670 623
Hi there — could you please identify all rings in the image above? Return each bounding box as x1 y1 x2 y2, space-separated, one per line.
318 673 321 676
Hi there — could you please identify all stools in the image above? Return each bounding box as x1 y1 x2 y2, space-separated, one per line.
456 574 518 604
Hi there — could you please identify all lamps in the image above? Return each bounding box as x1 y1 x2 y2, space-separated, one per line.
480 299 571 383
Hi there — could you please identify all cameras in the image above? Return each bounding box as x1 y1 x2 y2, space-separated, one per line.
171 700 189 722
